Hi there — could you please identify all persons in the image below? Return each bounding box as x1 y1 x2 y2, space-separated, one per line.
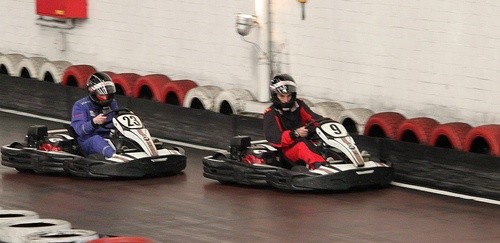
264 74 333 172
70 72 120 159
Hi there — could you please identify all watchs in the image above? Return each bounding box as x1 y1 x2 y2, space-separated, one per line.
294 130 300 138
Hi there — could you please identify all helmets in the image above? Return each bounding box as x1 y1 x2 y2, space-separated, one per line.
86 72 116 110
270 73 297 110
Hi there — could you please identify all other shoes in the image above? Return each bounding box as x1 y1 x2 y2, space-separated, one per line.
154 139 162 149
361 151 370 162
120 146 128 155
326 157 335 162
